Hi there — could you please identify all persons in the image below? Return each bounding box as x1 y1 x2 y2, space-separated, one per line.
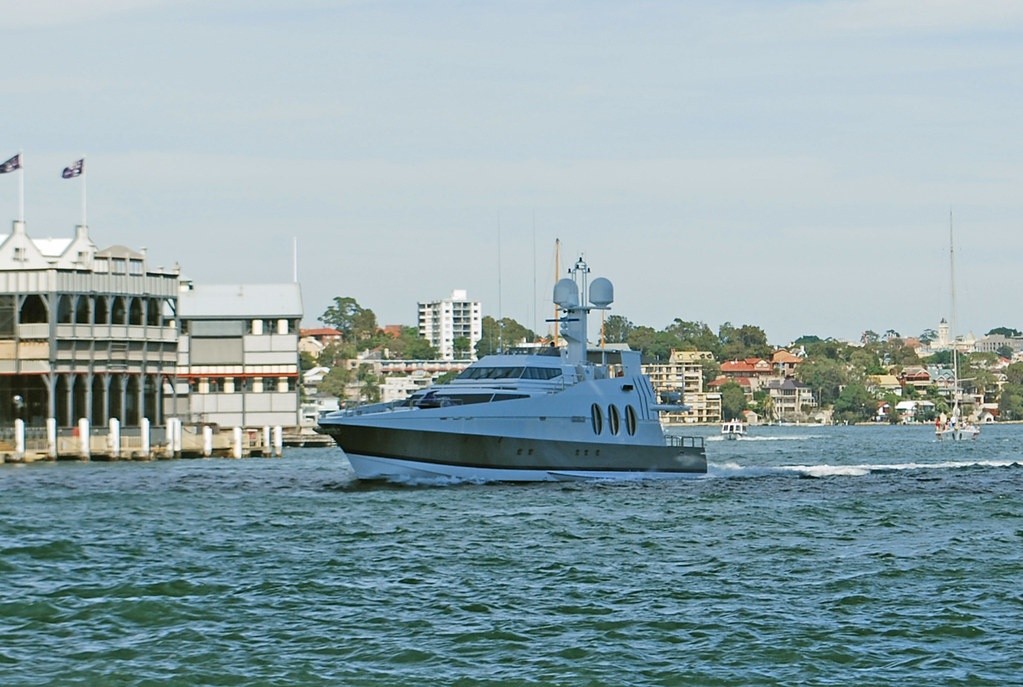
545 341 558 355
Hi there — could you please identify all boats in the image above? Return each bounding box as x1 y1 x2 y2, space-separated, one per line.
313 250 709 483
721 421 749 440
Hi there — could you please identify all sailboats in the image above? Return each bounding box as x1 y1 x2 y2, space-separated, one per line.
934 209 982 441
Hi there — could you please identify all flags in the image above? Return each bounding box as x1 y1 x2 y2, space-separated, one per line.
61 158 83 179
0 154 20 173
